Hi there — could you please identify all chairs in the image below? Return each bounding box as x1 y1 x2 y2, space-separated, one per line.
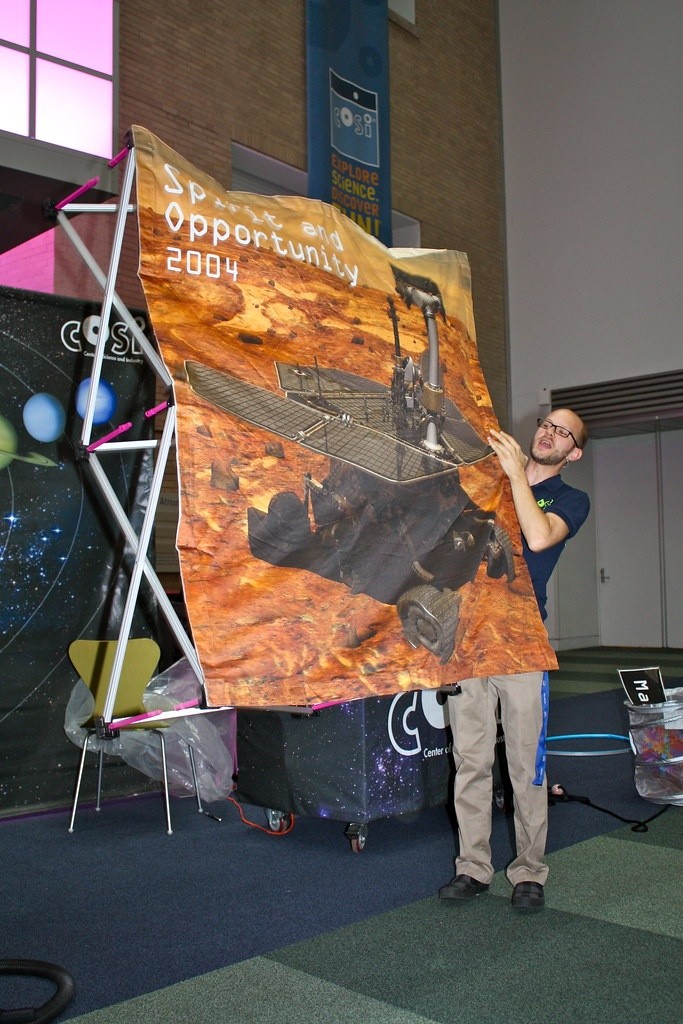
68 636 204 834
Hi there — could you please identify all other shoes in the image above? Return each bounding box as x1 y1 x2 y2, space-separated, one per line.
439 875 490 899
510 881 544 908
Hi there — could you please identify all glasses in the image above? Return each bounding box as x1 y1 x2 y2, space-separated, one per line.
536 417 580 448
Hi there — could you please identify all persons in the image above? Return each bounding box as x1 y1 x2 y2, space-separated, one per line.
438 408 590 910
